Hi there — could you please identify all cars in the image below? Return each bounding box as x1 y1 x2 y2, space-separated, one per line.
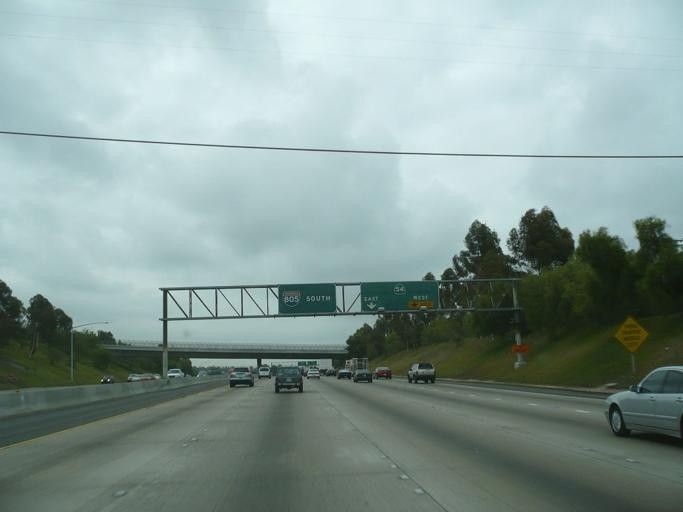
602 365 682 446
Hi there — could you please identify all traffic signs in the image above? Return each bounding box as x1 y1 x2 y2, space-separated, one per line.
276 282 338 315
360 280 439 313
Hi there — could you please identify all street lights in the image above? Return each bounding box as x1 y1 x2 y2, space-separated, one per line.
70 320 110 384
